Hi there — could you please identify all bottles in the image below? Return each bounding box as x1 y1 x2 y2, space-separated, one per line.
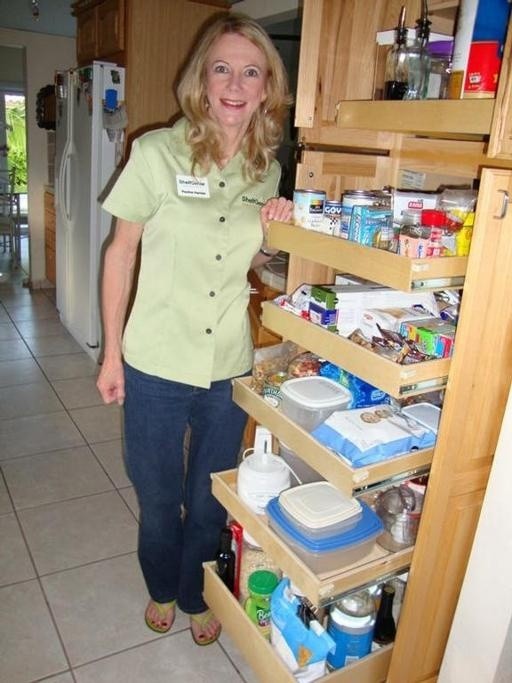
215 528 235 592
238 528 282 599
247 570 279 643
401 0 432 100
370 586 395 651
383 5 409 100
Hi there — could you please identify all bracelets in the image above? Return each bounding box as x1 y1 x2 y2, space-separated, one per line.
260 249 278 259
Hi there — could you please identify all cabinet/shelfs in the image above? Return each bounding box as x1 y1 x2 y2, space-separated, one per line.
202 1 512 683
69 0 226 163
43 192 55 284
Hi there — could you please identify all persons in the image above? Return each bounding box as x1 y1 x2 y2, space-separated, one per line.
93 11 293 645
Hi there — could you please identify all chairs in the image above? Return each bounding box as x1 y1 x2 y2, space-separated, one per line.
0 168 17 268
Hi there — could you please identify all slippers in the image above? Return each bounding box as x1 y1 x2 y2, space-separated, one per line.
190 610 221 645
144 600 178 632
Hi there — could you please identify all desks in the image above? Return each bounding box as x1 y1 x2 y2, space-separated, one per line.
0 193 21 264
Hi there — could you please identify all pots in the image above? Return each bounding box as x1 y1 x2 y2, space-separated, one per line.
376 485 425 553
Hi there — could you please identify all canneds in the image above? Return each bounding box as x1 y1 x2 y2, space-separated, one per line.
292 189 391 240
326 603 377 673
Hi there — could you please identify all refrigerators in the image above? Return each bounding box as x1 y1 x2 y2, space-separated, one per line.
55 61 124 363
